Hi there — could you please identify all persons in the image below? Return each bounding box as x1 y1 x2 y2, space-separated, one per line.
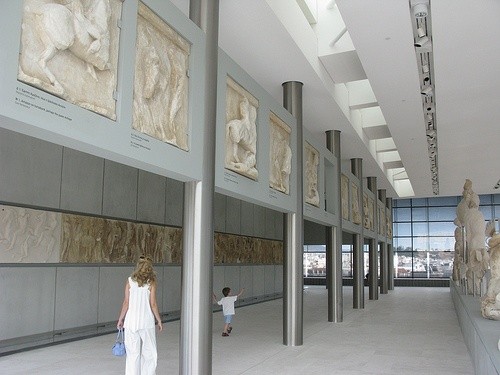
453 179 500 320
215 287 245 336
117 255 162 375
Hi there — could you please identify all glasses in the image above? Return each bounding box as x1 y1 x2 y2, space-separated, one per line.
140 256 152 262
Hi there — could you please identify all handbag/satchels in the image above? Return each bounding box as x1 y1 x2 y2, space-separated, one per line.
112 328 125 356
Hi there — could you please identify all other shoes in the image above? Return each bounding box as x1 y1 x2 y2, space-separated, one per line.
227 327 232 333
222 332 229 336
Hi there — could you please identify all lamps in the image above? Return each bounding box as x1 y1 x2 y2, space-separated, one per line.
413 26 430 51
424 106 439 198
420 80 433 101
411 0 430 20
